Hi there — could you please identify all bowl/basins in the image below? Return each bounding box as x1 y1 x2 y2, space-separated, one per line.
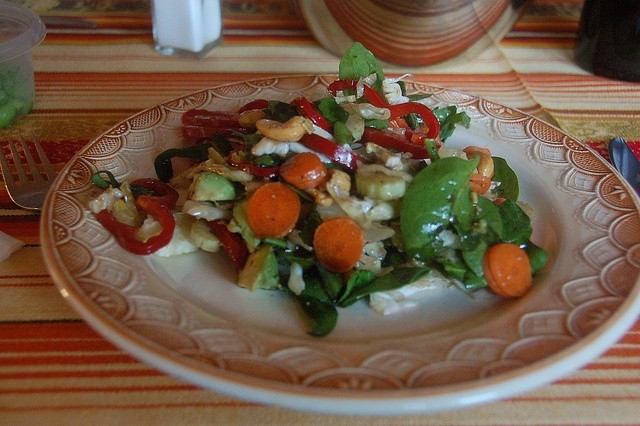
0 2 47 128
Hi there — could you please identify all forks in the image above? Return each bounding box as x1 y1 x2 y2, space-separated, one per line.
0 129 57 211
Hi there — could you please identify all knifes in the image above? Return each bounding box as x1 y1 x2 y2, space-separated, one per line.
607 137 639 195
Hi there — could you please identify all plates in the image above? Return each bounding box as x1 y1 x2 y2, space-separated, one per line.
37 72 640 418
